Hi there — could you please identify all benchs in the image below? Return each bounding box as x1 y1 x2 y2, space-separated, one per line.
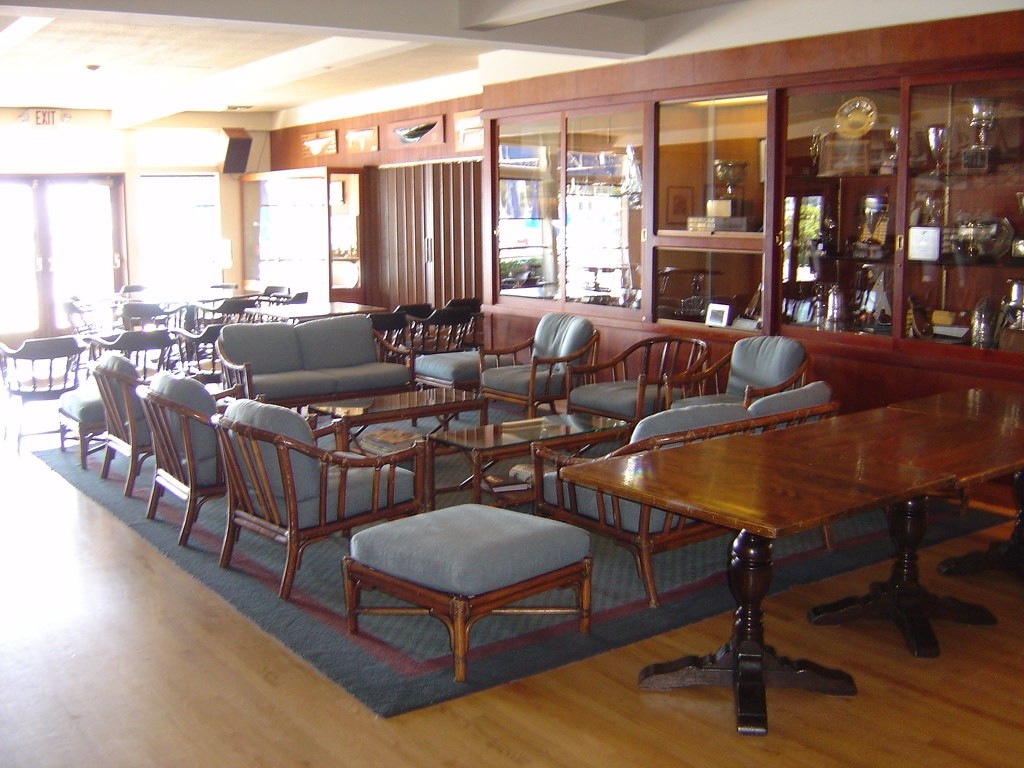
214 312 417 427
530 379 842 610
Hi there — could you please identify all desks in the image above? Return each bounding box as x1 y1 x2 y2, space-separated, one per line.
563 388 1023 736
196 288 259 306
245 301 389 321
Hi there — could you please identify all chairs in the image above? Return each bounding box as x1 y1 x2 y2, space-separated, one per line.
0 284 809 603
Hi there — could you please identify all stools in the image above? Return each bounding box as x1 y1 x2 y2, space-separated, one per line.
58 388 106 469
340 503 593 682
413 349 523 420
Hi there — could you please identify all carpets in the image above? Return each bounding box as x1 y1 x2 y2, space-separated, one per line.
31 400 1014 717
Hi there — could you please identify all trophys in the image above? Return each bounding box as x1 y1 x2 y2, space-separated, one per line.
668 86 1024 359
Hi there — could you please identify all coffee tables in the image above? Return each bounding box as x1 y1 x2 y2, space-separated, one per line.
423 411 630 511
308 384 489 454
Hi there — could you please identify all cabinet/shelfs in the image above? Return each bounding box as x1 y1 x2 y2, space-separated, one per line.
773 51 1022 363
480 90 768 332
237 166 366 304
